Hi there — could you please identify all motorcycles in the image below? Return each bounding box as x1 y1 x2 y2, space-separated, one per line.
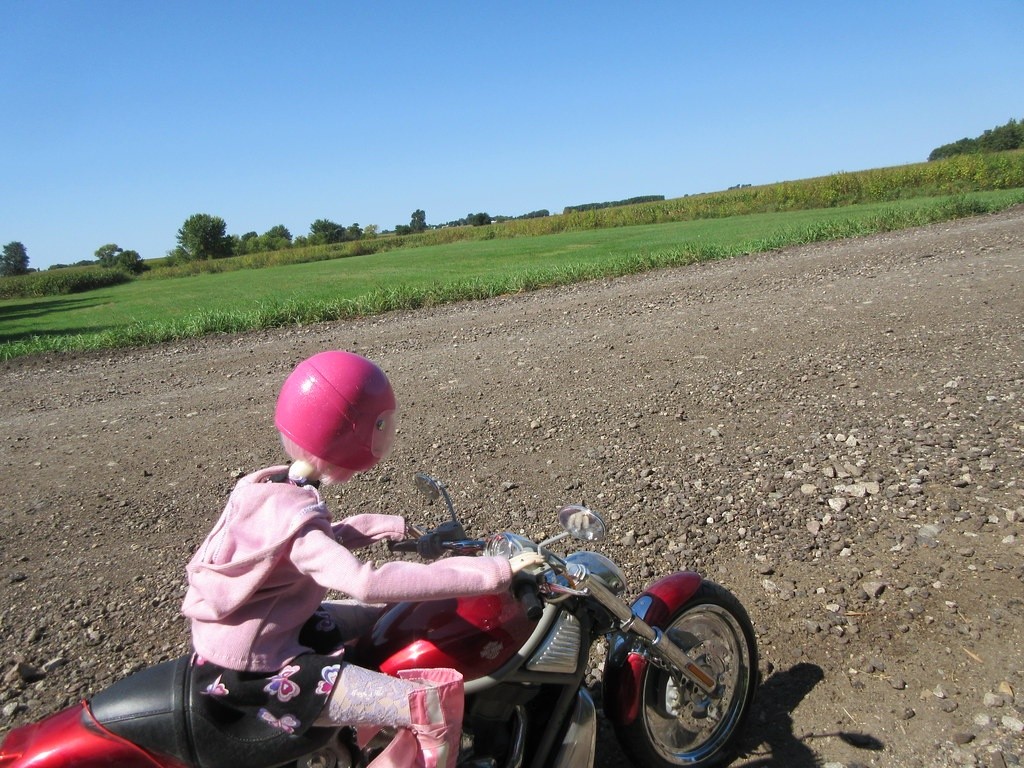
0 481 756 768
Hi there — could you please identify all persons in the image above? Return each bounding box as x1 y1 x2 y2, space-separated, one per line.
179 349 550 768
